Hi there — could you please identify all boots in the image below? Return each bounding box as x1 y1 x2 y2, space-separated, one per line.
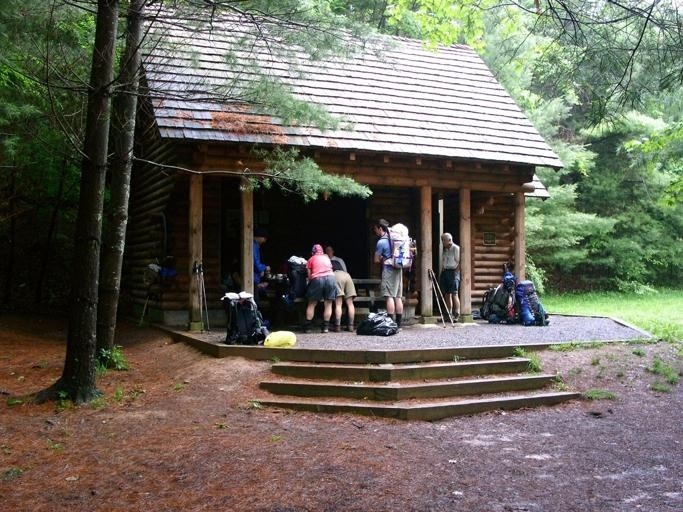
322 321 329 332
348 326 354 332
387 314 401 328
438 313 460 322
334 325 341 332
304 320 312 333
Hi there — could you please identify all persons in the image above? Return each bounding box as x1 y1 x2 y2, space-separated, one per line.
252 233 270 295
437 232 460 322
374 218 404 327
332 260 357 333
306 244 336 332
325 247 347 270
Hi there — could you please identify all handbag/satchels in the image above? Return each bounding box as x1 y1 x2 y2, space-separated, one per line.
388 223 417 272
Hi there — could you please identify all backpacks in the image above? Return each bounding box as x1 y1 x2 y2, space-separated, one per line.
480 272 550 325
286 256 308 297
216 292 270 345
357 312 398 335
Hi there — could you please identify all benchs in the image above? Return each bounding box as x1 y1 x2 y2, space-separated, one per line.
261 289 406 327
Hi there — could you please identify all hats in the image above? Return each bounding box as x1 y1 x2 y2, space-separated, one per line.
312 244 323 255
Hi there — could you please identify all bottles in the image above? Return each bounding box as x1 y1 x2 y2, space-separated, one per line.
255 317 261 328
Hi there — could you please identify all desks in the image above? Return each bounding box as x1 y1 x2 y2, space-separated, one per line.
267 277 381 327
